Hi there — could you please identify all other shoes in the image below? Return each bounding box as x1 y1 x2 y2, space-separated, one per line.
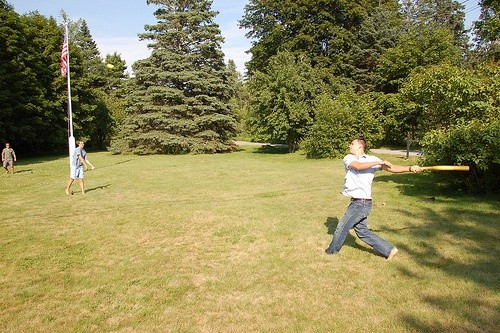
388 246 398 259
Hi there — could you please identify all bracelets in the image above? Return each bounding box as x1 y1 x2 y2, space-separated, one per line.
409 165 412 172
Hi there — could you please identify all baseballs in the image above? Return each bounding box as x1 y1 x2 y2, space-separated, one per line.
107 63 112 68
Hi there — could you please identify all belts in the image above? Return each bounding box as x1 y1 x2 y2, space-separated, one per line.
351 197 372 201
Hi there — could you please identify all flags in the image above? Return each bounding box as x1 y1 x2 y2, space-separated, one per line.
61 31 68 75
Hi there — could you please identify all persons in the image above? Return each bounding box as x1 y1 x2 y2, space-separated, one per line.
316 138 422 261
1 143 17 175
65 139 95 196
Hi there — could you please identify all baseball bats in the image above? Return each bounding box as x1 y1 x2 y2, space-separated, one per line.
418 165 470 171
